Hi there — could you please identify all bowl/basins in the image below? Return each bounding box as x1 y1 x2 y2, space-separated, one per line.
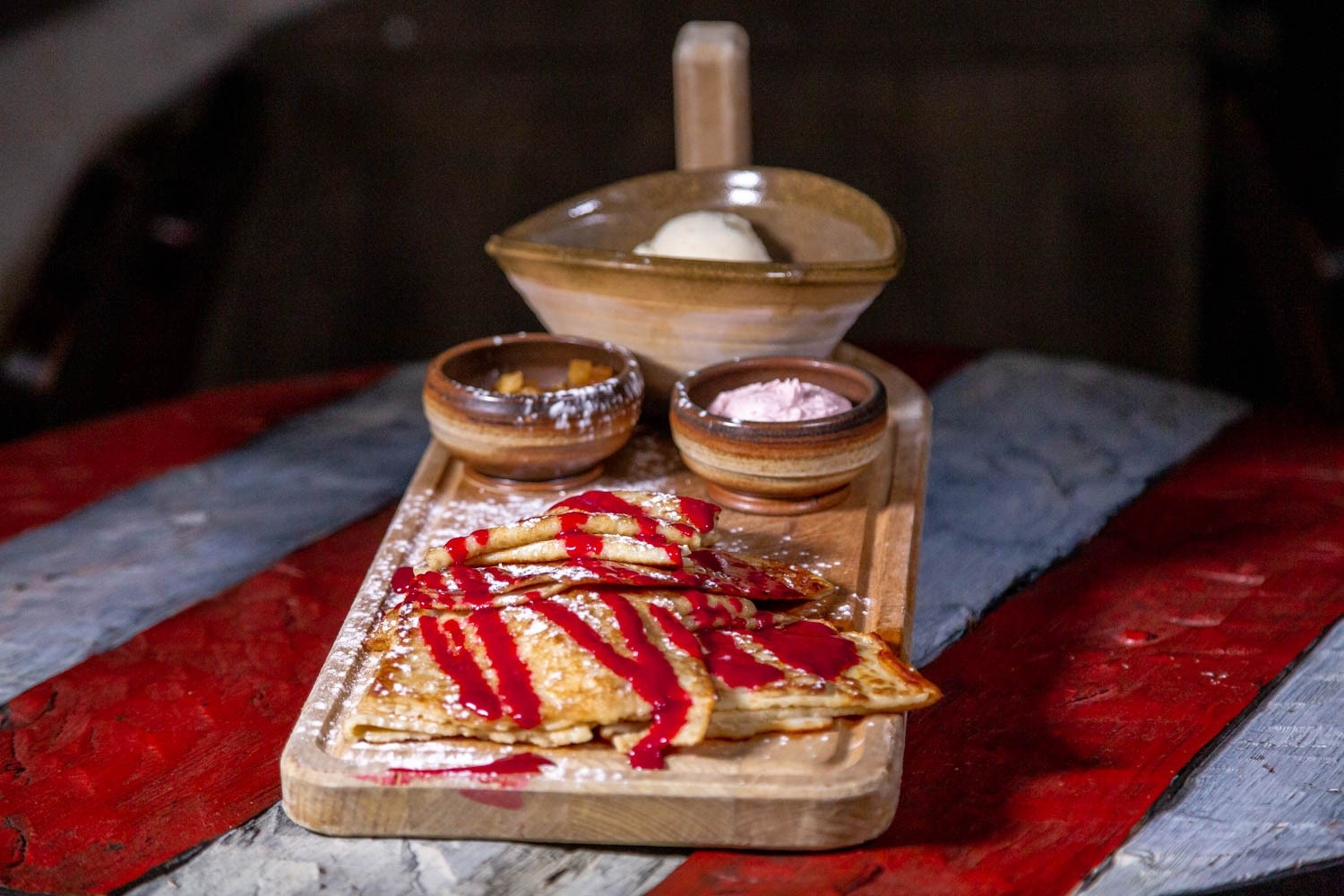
483 165 909 401
669 348 892 516
426 332 648 481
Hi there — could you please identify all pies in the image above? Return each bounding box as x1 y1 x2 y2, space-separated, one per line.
349 490 943 757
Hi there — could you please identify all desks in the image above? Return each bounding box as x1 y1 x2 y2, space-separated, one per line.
0 347 1344 896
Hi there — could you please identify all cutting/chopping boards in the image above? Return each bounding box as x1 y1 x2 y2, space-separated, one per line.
281 328 926 857
